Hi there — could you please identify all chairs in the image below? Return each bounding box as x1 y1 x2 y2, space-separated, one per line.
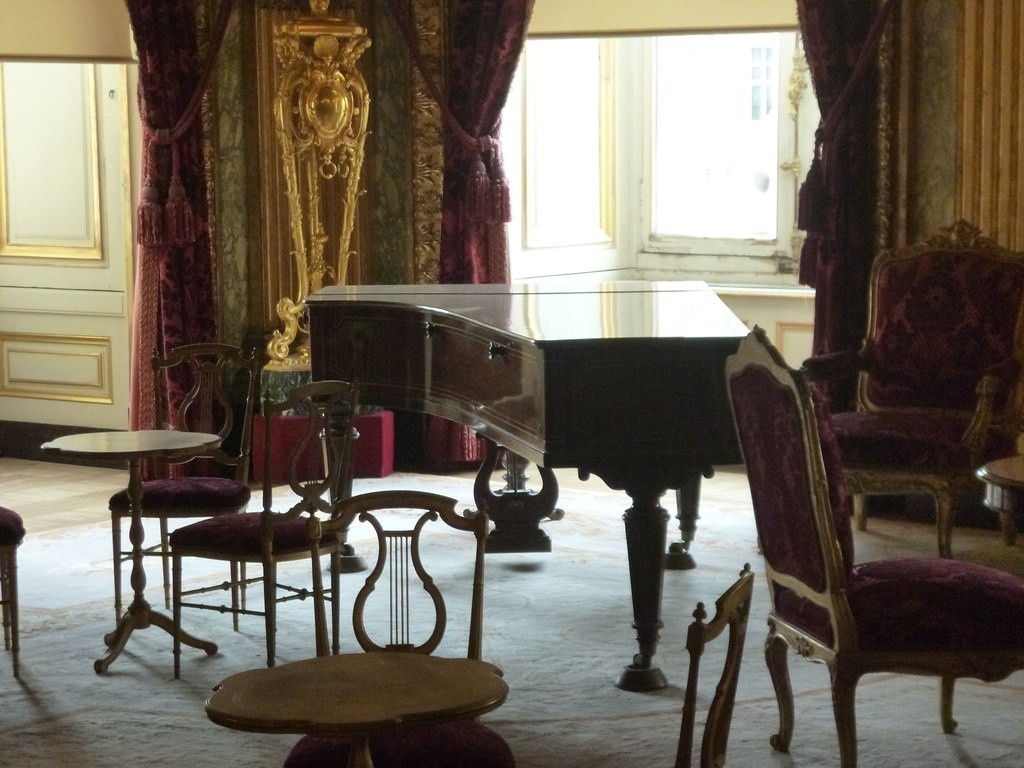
283 491 517 768
169 379 360 679
725 325 1024 768
799 220 1024 560
108 344 262 631
675 563 754 768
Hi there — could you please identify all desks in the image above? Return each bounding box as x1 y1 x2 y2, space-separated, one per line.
204 652 509 768
40 430 222 674
